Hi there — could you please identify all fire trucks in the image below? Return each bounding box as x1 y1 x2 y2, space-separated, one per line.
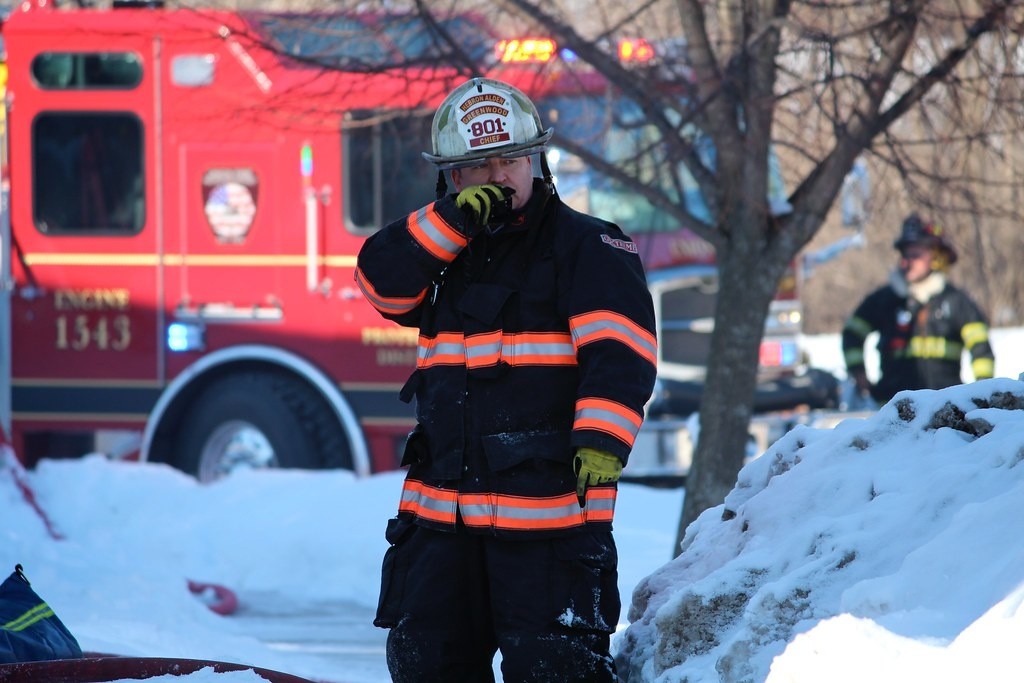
0 0 873 480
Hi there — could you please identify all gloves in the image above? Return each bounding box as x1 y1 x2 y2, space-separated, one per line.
572 448 623 508
455 184 514 231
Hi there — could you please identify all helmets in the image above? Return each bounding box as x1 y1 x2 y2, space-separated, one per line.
421 77 556 170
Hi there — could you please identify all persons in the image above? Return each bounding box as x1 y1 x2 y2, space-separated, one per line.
355 74 656 683
841 213 994 406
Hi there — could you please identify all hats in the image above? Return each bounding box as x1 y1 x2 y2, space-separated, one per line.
895 212 959 264
